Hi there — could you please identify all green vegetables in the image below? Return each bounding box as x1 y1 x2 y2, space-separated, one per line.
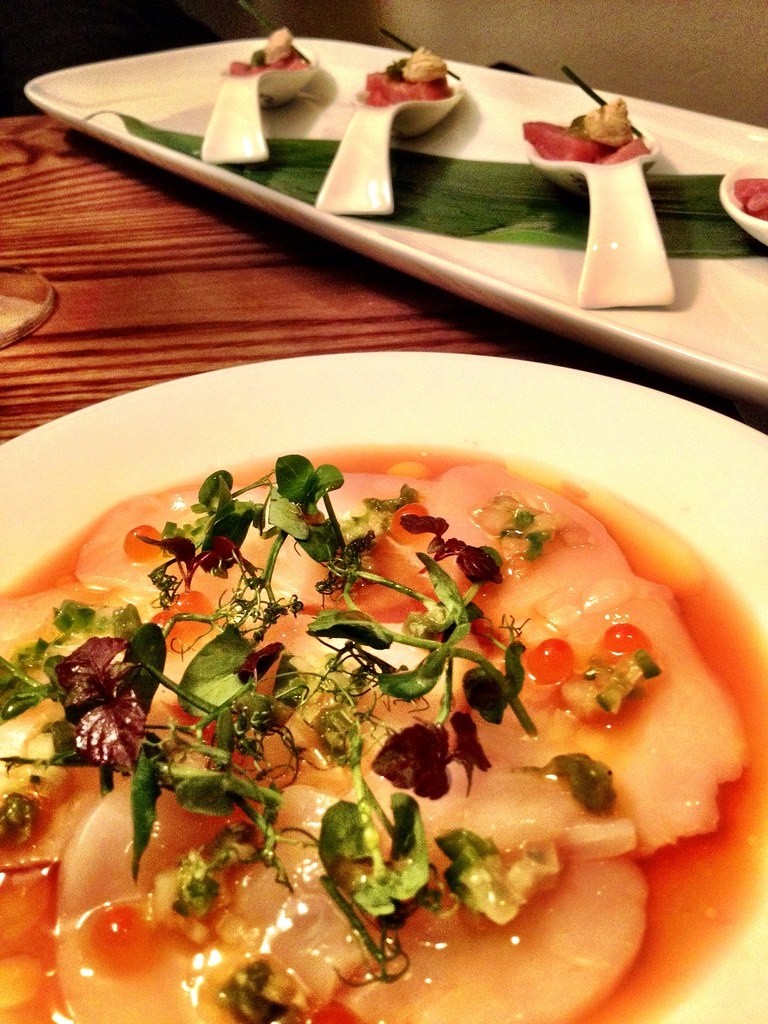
0 454 543 987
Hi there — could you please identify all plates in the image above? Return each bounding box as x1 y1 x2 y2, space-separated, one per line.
23 36 768 404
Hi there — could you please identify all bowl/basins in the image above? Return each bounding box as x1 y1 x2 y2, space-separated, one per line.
2 351 768 1024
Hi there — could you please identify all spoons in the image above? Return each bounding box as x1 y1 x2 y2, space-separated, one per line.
719 153 768 247
524 102 675 309
201 42 318 166
315 58 464 216
